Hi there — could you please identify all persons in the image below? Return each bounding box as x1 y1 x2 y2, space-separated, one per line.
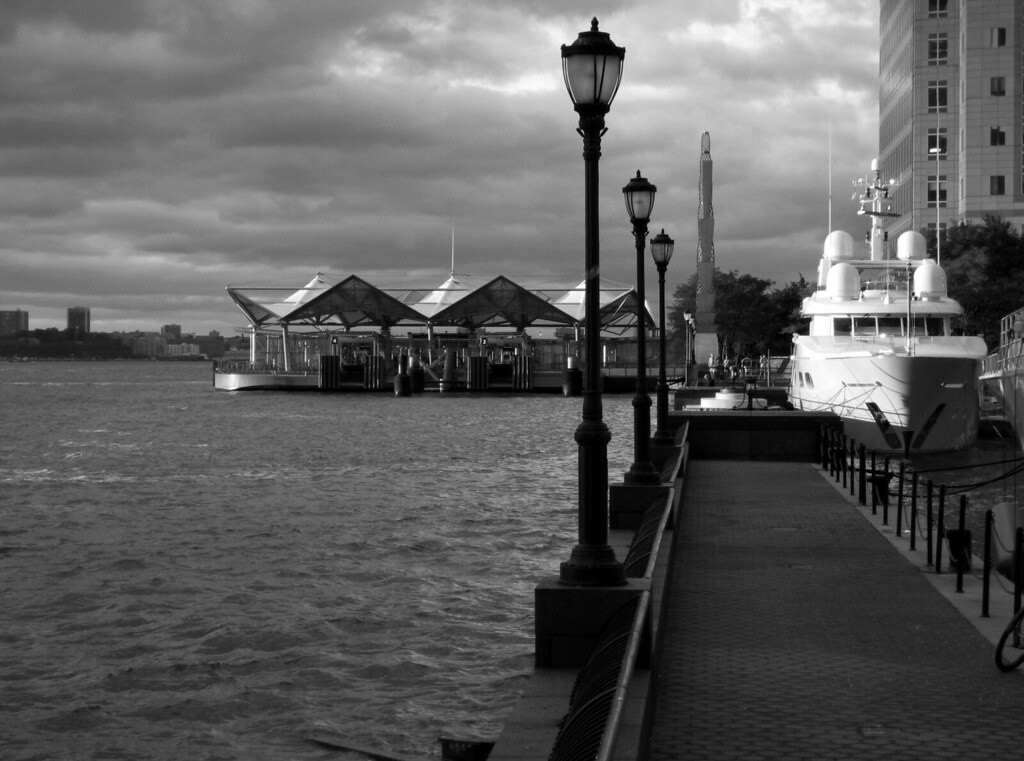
733 353 740 365
708 353 718 380
723 355 730 373
759 354 768 379
646 352 657 365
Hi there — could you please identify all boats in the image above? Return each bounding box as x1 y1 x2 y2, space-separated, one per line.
682 386 783 410
785 168 988 454
979 307 1024 453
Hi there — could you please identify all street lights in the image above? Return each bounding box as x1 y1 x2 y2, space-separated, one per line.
650 228 675 446
622 169 661 485
561 16 627 587
